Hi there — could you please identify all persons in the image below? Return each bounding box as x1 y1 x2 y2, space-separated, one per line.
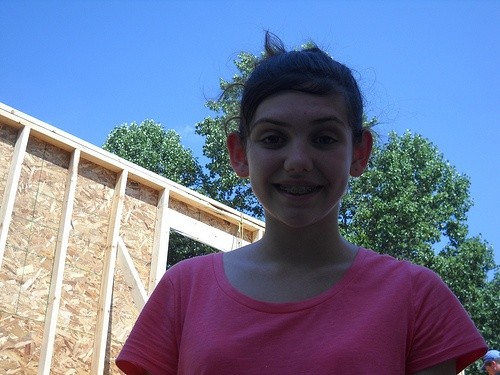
482 350 500 375
116 30 487 375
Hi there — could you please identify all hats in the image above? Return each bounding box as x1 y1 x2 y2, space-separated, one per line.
480 348 500 371
